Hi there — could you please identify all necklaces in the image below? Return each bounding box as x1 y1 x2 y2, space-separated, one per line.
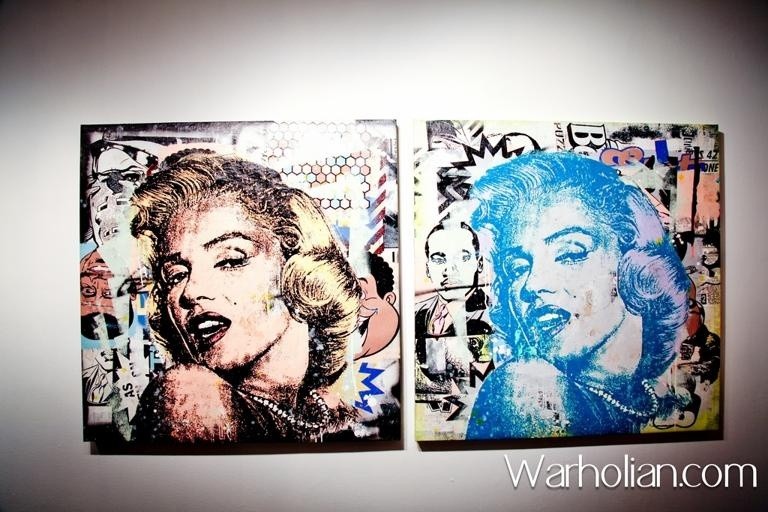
576 377 660 418
249 390 332 430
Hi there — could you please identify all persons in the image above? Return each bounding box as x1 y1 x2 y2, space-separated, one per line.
136 147 364 441
461 149 698 438
414 217 496 405
